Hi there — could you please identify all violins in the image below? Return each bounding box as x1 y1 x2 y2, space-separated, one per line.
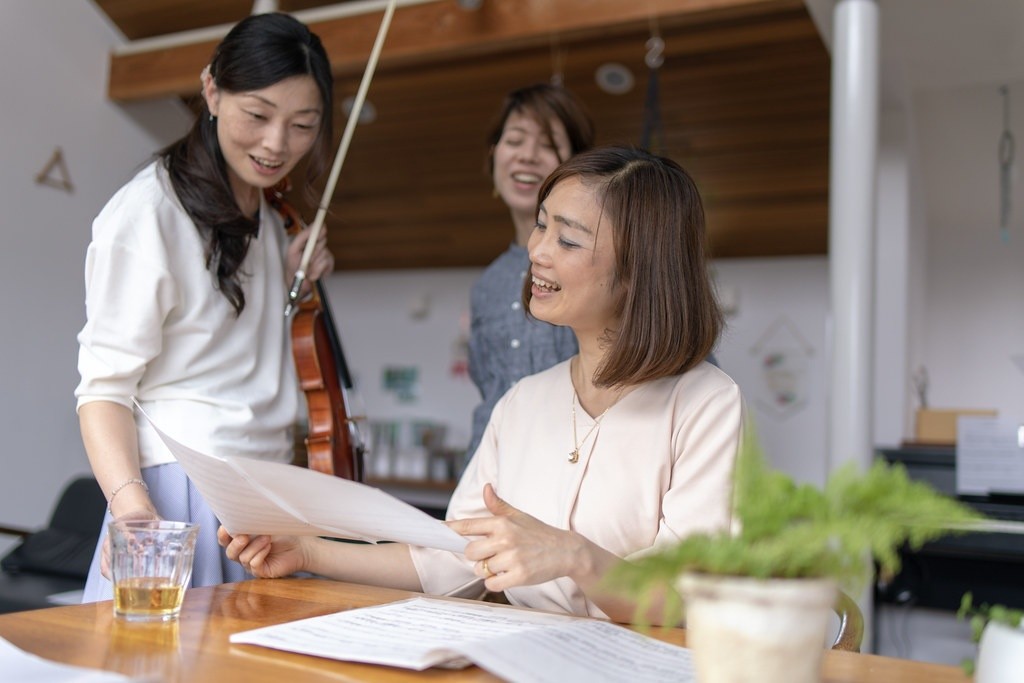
271 192 368 482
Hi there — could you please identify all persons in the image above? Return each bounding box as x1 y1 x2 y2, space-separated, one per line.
469 88 720 449
74 13 334 604
216 146 752 626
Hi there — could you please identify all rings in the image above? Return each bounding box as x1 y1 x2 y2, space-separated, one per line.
482 558 497 576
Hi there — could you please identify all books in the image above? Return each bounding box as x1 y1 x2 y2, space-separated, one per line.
229 598 696 683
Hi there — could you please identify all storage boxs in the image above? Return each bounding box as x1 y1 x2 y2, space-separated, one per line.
914 407 998 444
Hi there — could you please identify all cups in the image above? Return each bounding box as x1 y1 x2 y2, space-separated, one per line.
106 520 202 623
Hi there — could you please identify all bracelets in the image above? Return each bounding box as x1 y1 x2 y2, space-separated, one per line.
108 478 150 516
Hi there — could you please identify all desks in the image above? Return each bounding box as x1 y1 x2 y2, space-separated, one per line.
364 475 455 513
0 576 978 682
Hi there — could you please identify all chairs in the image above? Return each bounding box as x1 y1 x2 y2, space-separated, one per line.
0 475 107 613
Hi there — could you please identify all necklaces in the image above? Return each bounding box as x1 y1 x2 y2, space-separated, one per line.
567 387 623 463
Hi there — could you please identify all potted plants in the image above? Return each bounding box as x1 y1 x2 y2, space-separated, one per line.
598 437 972 683
955 594 1024 681
